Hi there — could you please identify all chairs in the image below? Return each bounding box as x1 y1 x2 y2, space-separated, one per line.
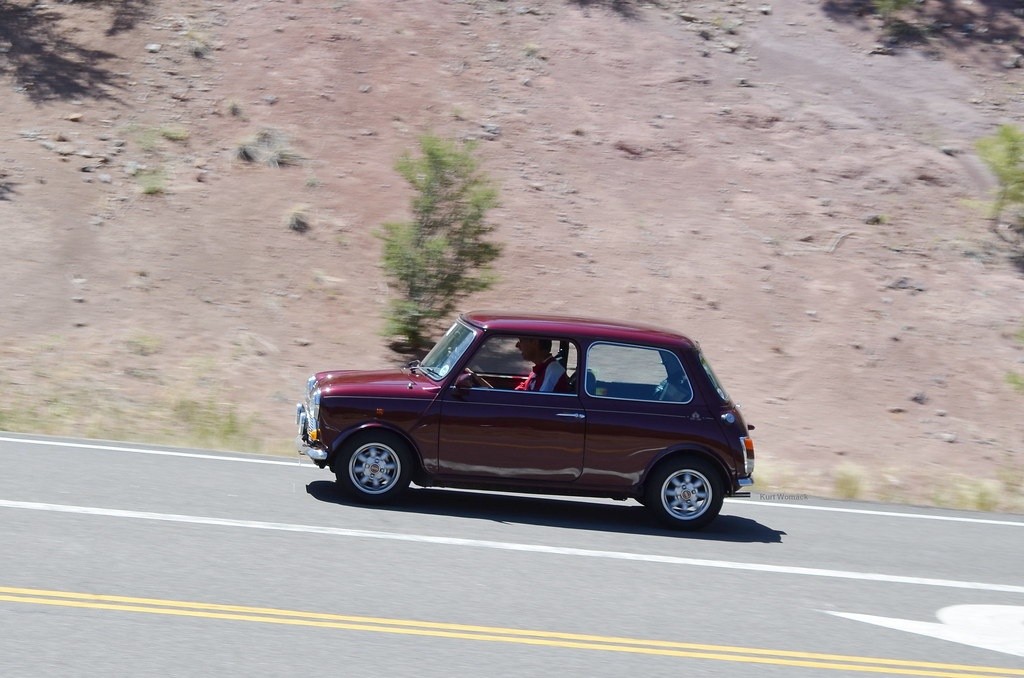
570 367 596 396
554 353 566 370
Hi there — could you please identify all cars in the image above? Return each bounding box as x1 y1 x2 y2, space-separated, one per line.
296 308 756 529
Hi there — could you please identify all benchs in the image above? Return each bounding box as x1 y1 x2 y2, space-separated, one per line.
651 376 691 402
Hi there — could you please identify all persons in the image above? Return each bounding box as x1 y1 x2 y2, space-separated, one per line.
515 337 569 393
